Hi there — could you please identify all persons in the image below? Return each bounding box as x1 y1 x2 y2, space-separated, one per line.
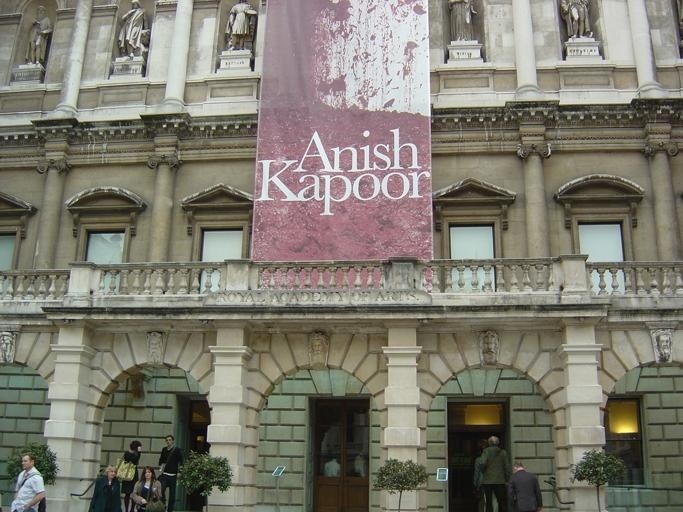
128 466 162 512
473 446 499 512
115 0 148 56
24 5 52 65
509 461 544 511
8 453 45 511
158 434 183 511
447 1 478 42
558 1 593 39
120 440 142 512
223 1 259 53
478 435 511 512
324 449 339 477
87 464 123 511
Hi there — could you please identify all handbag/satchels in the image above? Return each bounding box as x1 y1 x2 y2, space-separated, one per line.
38 496 47 512
146 497 166 512
115 458 137 482
